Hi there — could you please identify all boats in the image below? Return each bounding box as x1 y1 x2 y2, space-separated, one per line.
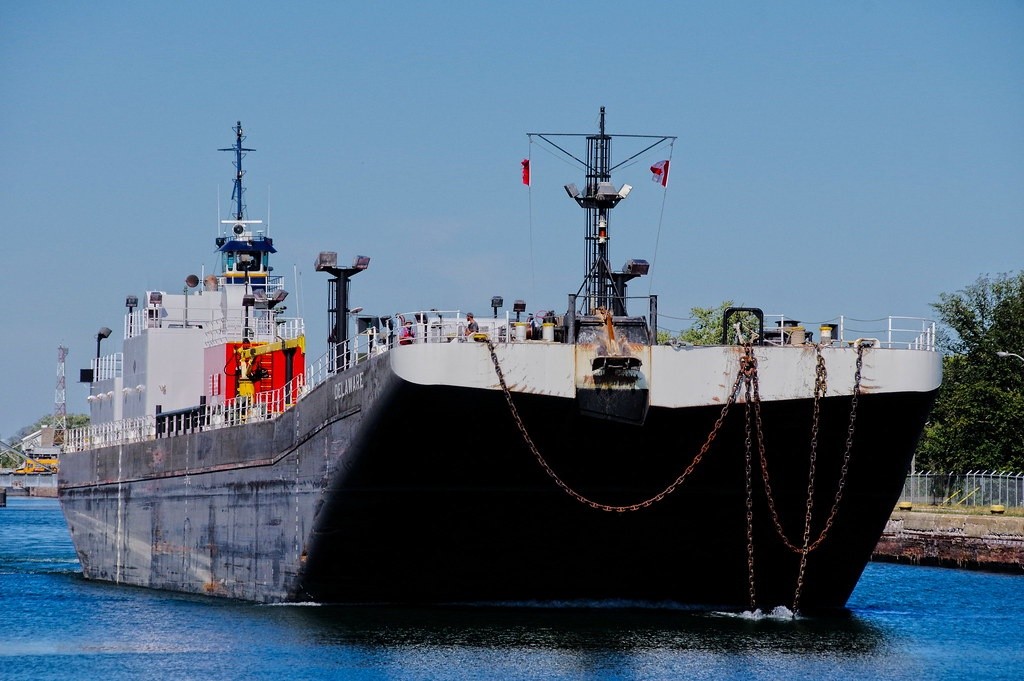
56 104 940 616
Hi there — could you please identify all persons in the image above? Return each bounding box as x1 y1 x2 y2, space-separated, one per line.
399 321 415 345
461 312 480 343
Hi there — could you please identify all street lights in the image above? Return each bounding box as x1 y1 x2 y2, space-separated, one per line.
997 351 1024 361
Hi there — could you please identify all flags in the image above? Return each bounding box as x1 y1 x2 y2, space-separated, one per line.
650 160 670 188
520 158 531 186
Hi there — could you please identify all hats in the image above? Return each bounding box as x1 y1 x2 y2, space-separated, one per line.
404 319 413 324
